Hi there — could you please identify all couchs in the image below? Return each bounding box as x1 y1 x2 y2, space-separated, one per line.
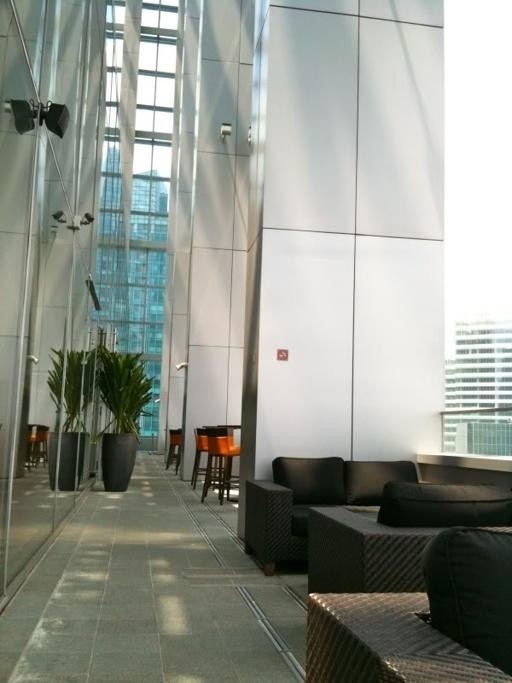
306 506 512 600
246 479 428 575
305 591 511 683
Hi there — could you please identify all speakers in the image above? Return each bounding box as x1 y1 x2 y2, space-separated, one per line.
10 99 35 135
45 103 70 138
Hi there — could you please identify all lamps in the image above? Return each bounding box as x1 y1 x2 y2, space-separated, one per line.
7 95 39 136
39 99 72 140
219 122 233 144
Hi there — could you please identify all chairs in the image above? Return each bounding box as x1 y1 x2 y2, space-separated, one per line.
26 418 51 469
165 423 240 506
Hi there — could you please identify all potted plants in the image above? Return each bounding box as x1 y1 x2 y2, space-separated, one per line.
41 346 100 490
100 343 161 493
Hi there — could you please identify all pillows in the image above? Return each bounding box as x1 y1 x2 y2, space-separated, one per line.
272 456 345 502
377 481 512 527
425 526 512 674
343 461 418 504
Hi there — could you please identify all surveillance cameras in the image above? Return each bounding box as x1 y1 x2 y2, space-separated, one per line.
85 213 94 223
53 210 64 220
